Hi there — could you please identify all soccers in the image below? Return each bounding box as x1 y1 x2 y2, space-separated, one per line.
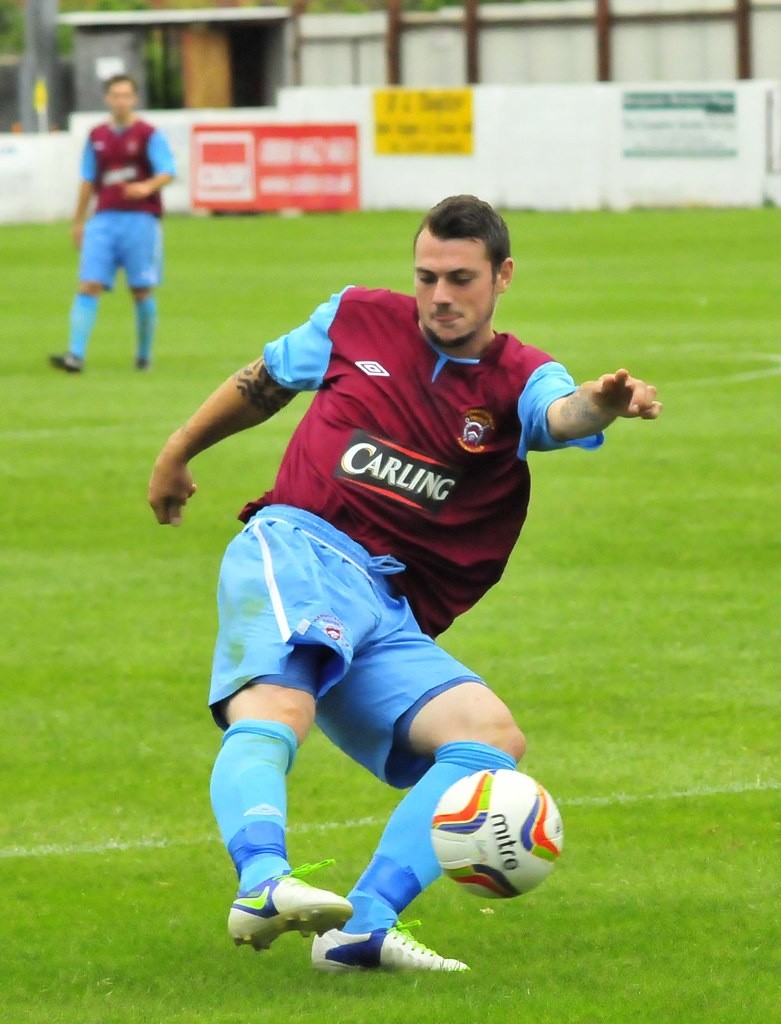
430 765 567 901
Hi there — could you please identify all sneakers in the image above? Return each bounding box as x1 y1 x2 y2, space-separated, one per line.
310 919 471 974
228 859 355 951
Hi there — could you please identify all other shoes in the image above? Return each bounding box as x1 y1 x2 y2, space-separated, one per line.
50 352 83 373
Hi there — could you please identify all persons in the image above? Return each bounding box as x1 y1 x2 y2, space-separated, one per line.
148 192 664 971
50 75 175 372
